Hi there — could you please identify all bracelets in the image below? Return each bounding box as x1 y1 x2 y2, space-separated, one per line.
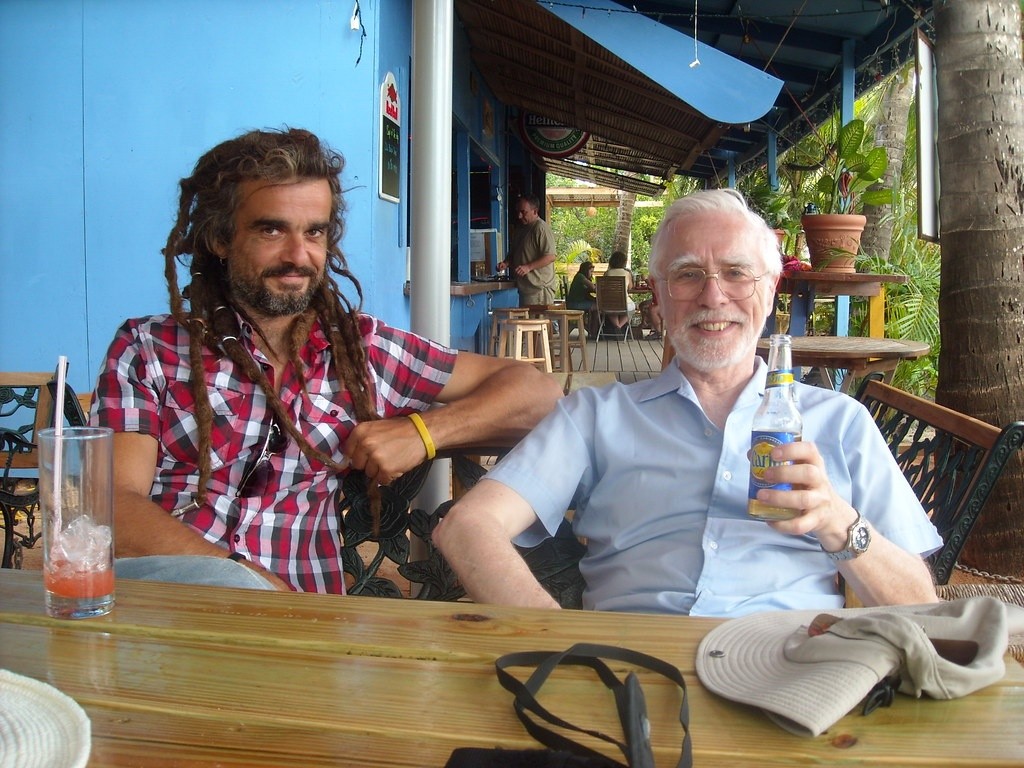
409 413 435 459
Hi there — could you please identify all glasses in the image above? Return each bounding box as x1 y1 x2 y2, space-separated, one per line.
235 410 289 499
651 266 771 301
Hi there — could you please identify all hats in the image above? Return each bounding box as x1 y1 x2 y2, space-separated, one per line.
695 592 1007 738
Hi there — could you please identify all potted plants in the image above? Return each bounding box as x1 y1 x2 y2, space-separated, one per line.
801 121 889 274
750 186 791 255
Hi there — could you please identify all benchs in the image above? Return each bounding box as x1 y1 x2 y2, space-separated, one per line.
47 373 1024 606
1 364 69 569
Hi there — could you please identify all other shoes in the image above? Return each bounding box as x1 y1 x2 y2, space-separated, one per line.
643 331 661 341
590 333 606 340
613 325 624 341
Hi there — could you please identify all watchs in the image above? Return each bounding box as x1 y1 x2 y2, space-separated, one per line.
819 505 873 561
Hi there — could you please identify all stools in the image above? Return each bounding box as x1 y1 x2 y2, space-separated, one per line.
490 306 588 374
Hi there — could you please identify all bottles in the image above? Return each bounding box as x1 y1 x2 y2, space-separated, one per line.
748 334 805 523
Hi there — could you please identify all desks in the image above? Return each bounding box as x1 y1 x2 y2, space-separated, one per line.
618 288 654 341
759 334 931 392
0 563 1023 768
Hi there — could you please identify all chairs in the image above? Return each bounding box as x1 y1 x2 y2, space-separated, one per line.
558 278 590 305
595 274 635 342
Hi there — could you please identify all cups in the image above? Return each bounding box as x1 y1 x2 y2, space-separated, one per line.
37 426 116 618
475 264 485 277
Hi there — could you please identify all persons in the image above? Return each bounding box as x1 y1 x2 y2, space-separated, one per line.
640 275 663 340
495 195 557 368
567 261 597 311
88 129 566 602
601 252 635 340
432 188 944 611
